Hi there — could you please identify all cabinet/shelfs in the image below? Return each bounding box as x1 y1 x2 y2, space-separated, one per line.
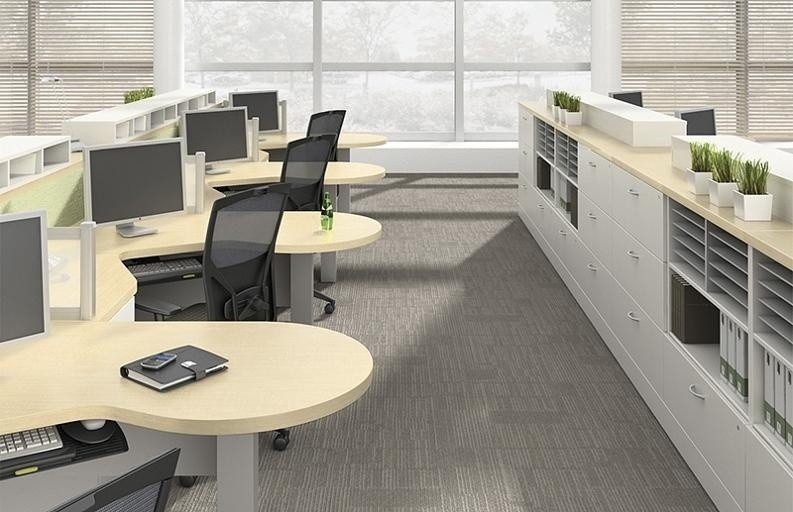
516 97 793 512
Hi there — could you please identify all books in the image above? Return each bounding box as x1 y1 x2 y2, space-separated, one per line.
120 344 229 390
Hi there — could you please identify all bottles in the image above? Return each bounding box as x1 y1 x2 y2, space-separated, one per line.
320 192 333 231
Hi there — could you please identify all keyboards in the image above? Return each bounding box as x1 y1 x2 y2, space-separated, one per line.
1 419 128 480
128 258 204 286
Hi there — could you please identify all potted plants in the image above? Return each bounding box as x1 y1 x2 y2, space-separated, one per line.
551 91 562 118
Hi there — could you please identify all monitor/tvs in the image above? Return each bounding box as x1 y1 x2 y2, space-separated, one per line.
609 91 642 108
0 209 51 347
180 106 249 175
675 106 716 135
229 90 282 140
82 136 188 238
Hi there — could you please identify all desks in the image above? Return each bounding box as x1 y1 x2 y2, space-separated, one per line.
204 161 385 283
258 130 386 213
1 320 374 511
45 210 381 324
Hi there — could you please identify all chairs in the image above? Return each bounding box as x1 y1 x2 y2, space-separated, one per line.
164 182 292 489
306 110 345 161
280 133 335 208
46 447 181 512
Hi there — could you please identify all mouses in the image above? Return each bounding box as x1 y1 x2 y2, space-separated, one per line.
81 420 106 431
